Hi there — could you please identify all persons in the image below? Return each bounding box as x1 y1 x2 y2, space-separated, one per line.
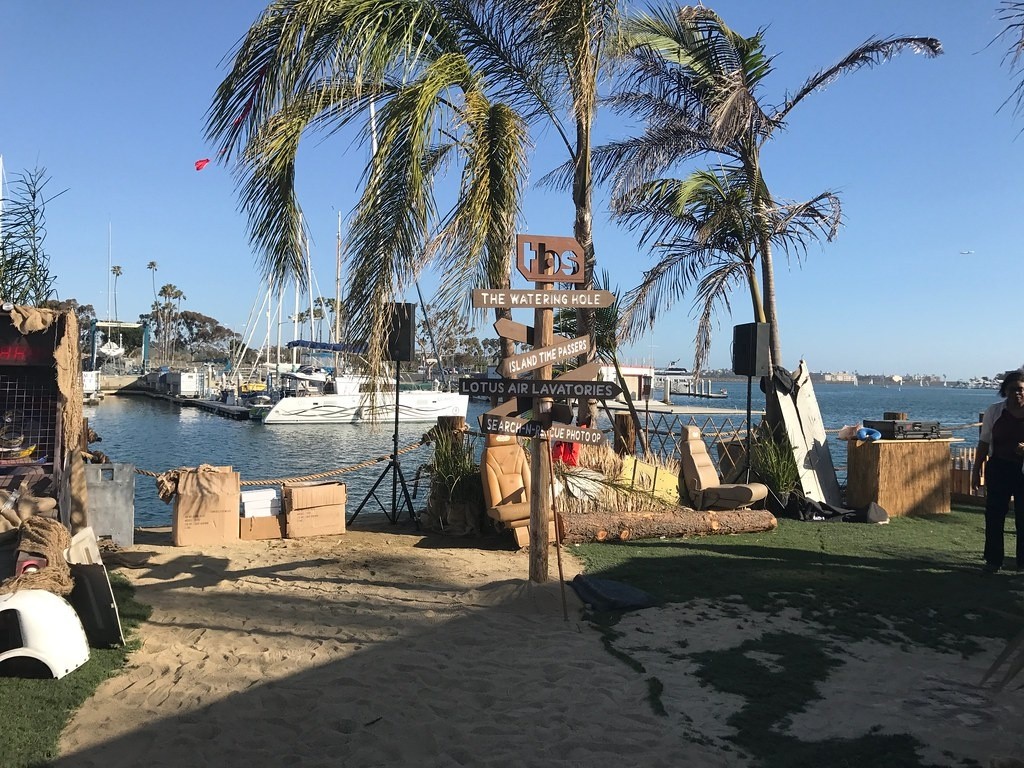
972 371 1024 573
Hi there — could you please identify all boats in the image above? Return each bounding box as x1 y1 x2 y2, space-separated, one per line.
652 358 695 394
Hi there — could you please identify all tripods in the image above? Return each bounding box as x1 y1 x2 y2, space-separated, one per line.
731 377 786 513
347 362 420 532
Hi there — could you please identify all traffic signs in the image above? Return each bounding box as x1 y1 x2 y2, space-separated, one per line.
458 378 625 396
495 334 590 379
470 288 617 309
482 414 605 445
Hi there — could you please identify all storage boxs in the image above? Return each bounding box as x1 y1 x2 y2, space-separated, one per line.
243 499 279 509
154 465 241 546
240 515 285 540
280 480 346 538
243 507 280 518
240 487 277 503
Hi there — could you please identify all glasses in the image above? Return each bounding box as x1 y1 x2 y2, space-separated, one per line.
1006 385 1024 392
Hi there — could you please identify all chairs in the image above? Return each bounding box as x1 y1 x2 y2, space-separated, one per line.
681 424 770 510
480 433 557 547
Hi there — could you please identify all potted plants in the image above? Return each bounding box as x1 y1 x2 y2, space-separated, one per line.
744 422 819 512
419 418 480 507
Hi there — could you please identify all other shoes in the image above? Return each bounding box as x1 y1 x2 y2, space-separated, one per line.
1015 556 1024 568
982 561 1003 572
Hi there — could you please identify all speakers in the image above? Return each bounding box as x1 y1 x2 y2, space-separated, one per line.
732 323 769 376
379 302 415 362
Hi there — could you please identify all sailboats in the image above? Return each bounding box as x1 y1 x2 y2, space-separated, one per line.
220 210 469 422
96 220 126 361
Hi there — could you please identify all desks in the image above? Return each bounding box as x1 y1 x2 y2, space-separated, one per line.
837 436 965 517
